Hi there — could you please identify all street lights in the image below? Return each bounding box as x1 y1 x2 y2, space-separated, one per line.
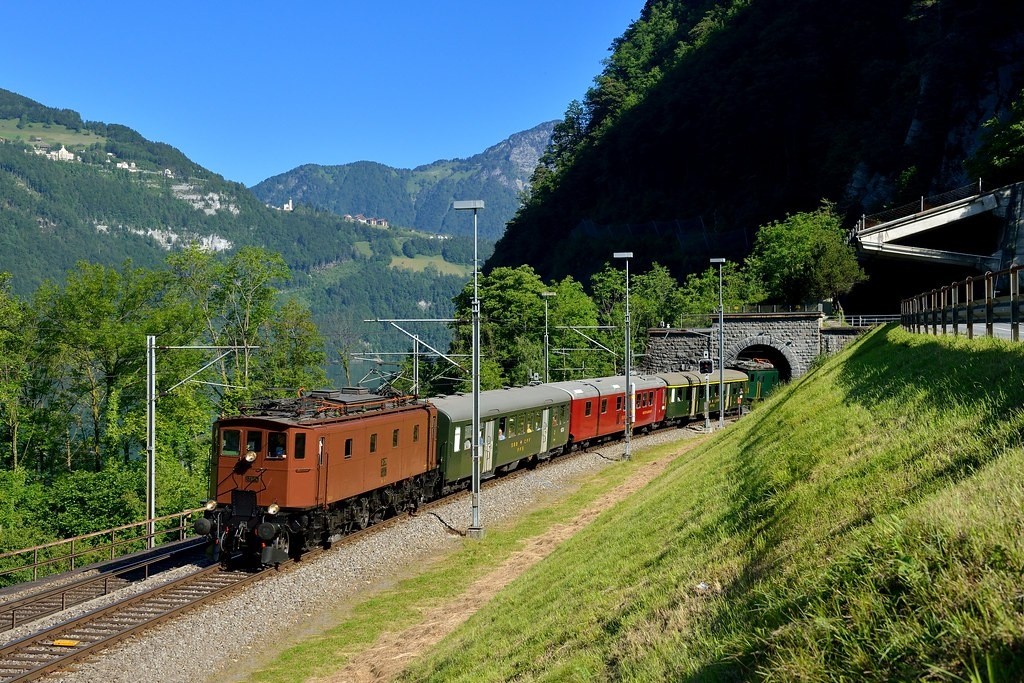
452 198 487 540
612 252 634 461
710 257 727 431
540 291 558 381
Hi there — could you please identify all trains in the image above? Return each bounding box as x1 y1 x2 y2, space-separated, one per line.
190 361 778 576
